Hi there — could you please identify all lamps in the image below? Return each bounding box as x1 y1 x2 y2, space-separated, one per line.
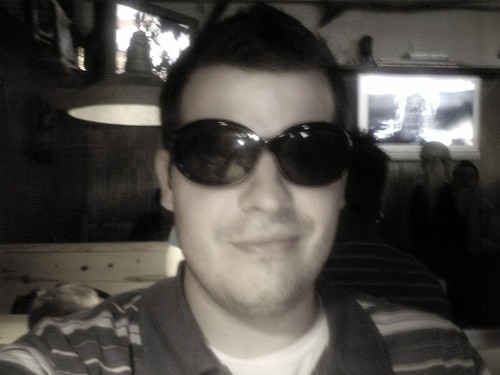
63 26 168 127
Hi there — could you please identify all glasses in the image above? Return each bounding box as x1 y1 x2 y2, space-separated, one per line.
166 116 355 187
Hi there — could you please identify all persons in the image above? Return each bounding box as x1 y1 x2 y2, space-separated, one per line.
27 280 100 331
318 133 454 322
392 140 498 285
0 6 493 375
376 93 431 147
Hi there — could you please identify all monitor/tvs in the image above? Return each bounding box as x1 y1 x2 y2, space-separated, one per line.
358 74 481 161
106 0 199 82
